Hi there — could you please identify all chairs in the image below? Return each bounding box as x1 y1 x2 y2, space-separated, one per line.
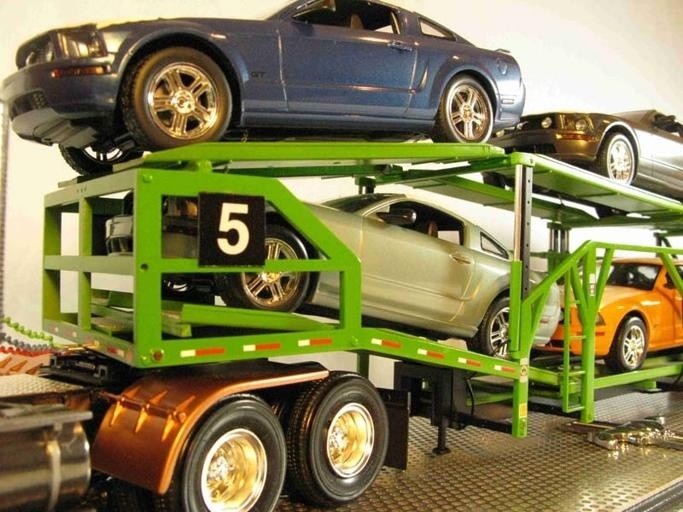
413 221 440 236
331 14 362 27
